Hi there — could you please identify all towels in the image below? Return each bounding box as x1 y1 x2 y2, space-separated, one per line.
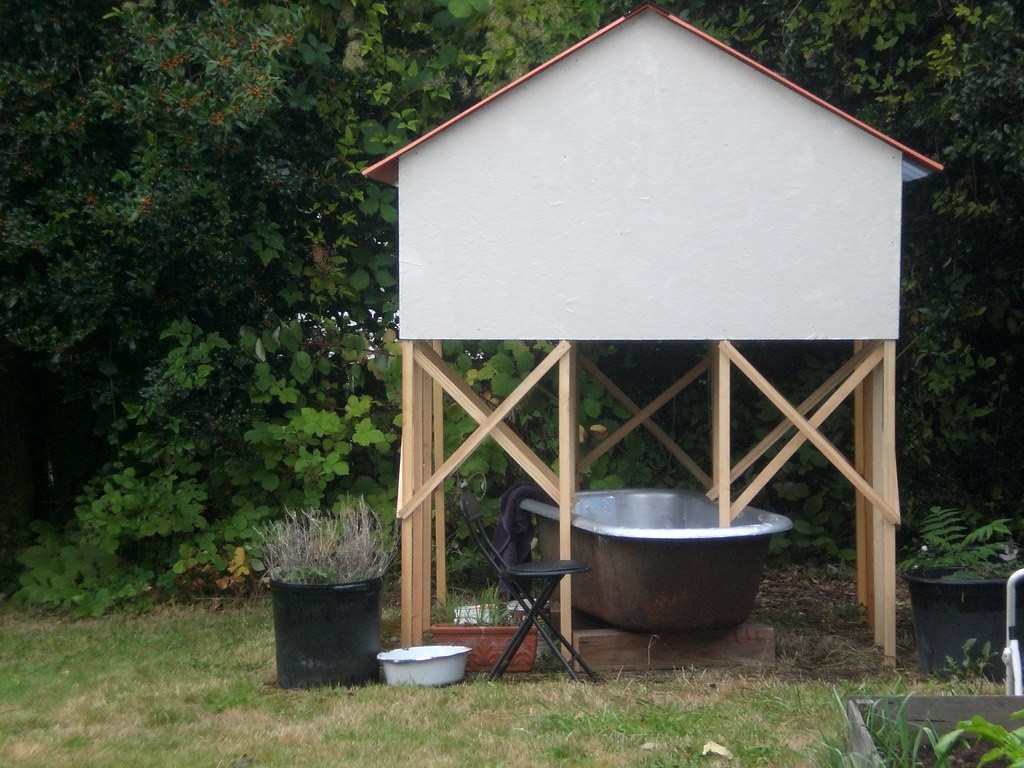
488 485 548 601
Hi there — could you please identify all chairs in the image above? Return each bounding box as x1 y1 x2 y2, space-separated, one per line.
459 491 599 688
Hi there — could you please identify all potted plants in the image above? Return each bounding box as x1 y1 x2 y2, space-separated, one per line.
255 493 402 691
846 694 1024 768
431 587 538 672
895 506 1024 685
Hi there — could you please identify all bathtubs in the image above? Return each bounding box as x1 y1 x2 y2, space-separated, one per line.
516 484 794 631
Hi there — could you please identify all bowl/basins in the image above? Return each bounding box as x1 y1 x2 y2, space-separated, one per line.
377 645 475 686
522 487 791 632
